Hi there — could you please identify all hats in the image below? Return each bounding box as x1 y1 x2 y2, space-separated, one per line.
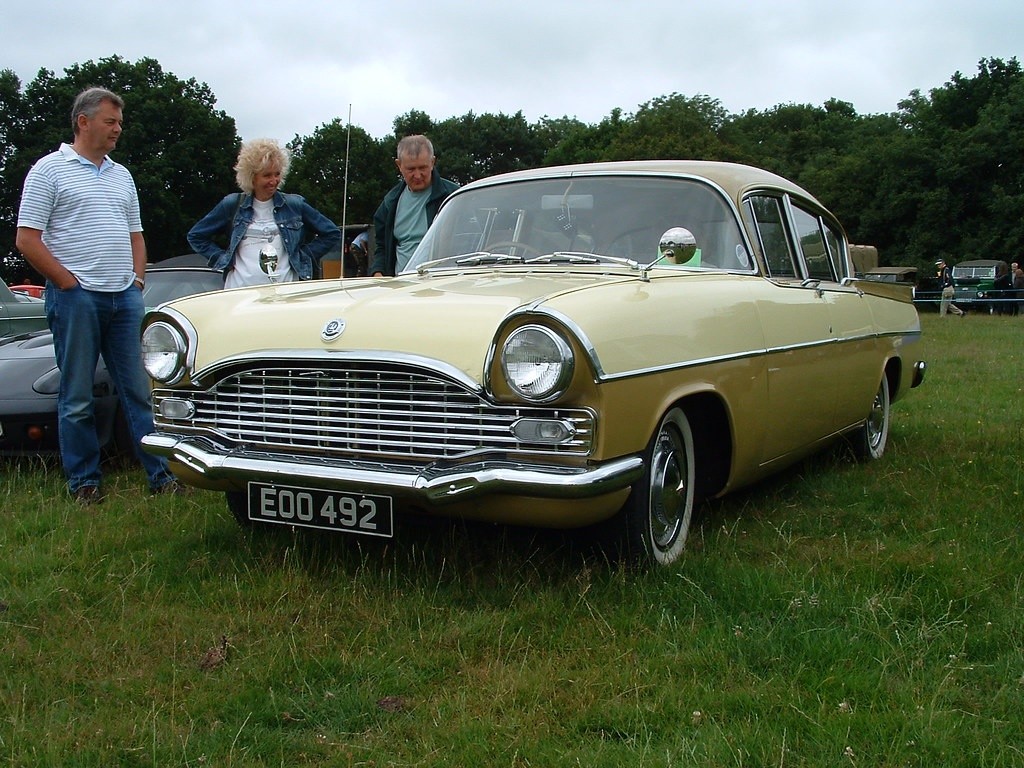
934 258 943 265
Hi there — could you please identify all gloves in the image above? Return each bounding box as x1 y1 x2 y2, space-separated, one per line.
940 284 945 289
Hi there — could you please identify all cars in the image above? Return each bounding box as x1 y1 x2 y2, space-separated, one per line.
0 253 224 459
950 258 1012 316
0 276 50 341
850 244 921 292
135 158 930 574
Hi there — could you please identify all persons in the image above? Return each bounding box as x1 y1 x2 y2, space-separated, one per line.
993 263 1024 317
15 88 191 503
348 231 368 277
186 139 342 291
935 259 967 318
366 136 482 276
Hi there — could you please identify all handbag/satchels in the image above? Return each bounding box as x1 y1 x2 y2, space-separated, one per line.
942 285 955 297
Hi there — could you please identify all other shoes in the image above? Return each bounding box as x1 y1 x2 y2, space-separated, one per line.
960 311 966 318
149 481 186 494
73 486 102 504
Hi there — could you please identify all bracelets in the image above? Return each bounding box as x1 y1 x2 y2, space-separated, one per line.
133 275 145 287
